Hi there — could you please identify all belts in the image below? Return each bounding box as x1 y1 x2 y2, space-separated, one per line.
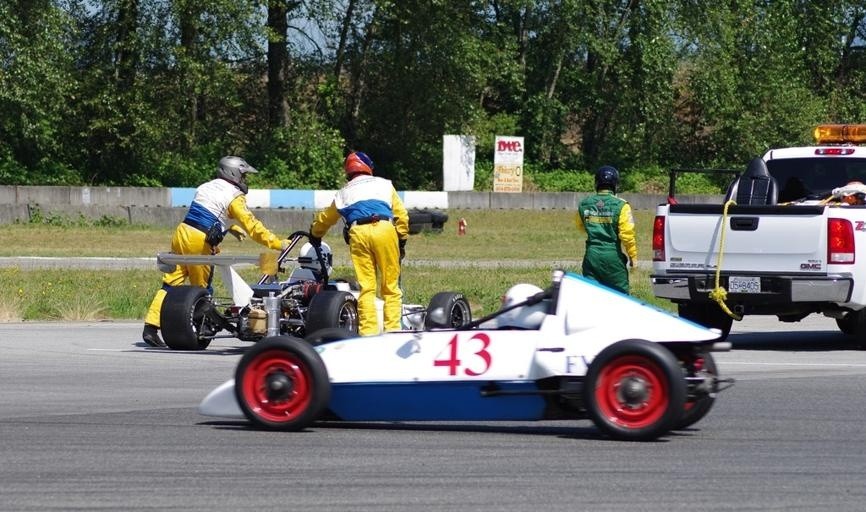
182 217 210 235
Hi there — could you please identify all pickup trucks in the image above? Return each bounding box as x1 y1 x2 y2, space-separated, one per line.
649 123 866 350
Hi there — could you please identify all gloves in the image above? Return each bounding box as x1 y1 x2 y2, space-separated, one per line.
308 225 321 248
228 224 248 243
398 236 407 262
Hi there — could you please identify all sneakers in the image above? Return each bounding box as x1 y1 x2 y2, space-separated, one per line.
141 323 167 346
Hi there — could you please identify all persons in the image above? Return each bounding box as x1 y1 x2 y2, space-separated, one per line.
308 152 410 337
284 241 332 284
142 156 295 347
575 166 638 295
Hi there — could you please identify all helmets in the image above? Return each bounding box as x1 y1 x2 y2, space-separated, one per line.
217 155 259 195
343 150 374 179
595 165 619 199
298 240 334 280
